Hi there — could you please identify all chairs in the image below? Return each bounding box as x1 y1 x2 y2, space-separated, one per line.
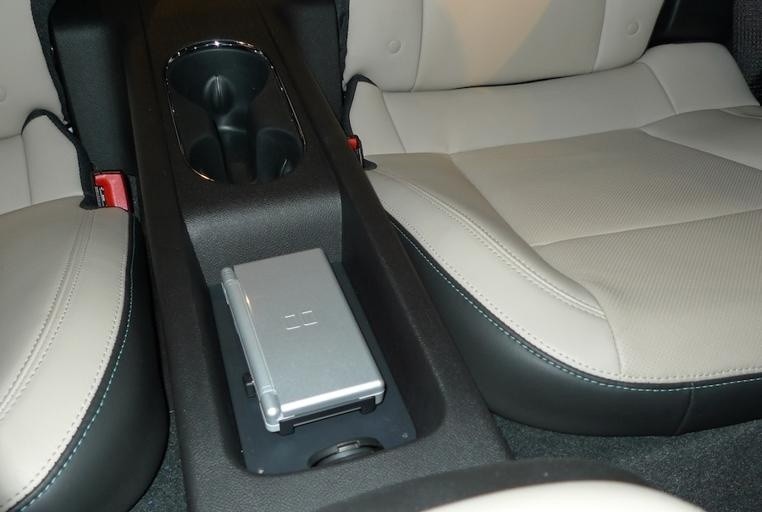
337 2 761 436
0 0 166 512
323 459 705 511
